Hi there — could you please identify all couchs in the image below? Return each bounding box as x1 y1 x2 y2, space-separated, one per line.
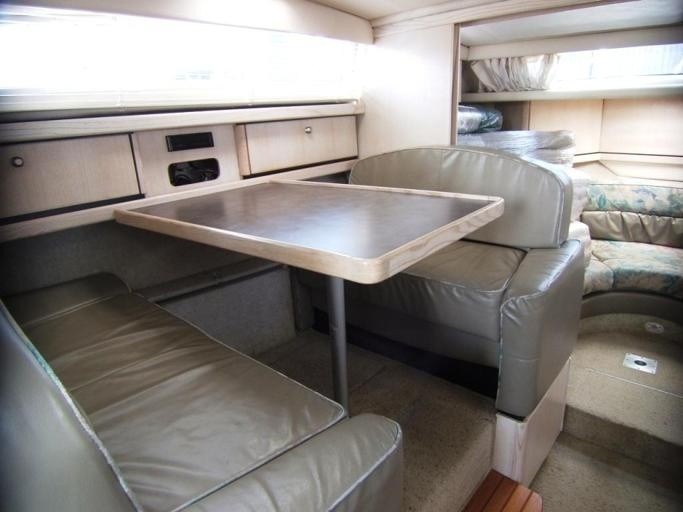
573 183 683 343
1 270 406 512
303 143 585 421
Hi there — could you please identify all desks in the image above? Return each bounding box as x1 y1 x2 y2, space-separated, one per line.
112 180 507 419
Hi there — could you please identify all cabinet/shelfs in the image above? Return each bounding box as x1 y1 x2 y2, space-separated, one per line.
232 116 360 178
1 133 146 228
